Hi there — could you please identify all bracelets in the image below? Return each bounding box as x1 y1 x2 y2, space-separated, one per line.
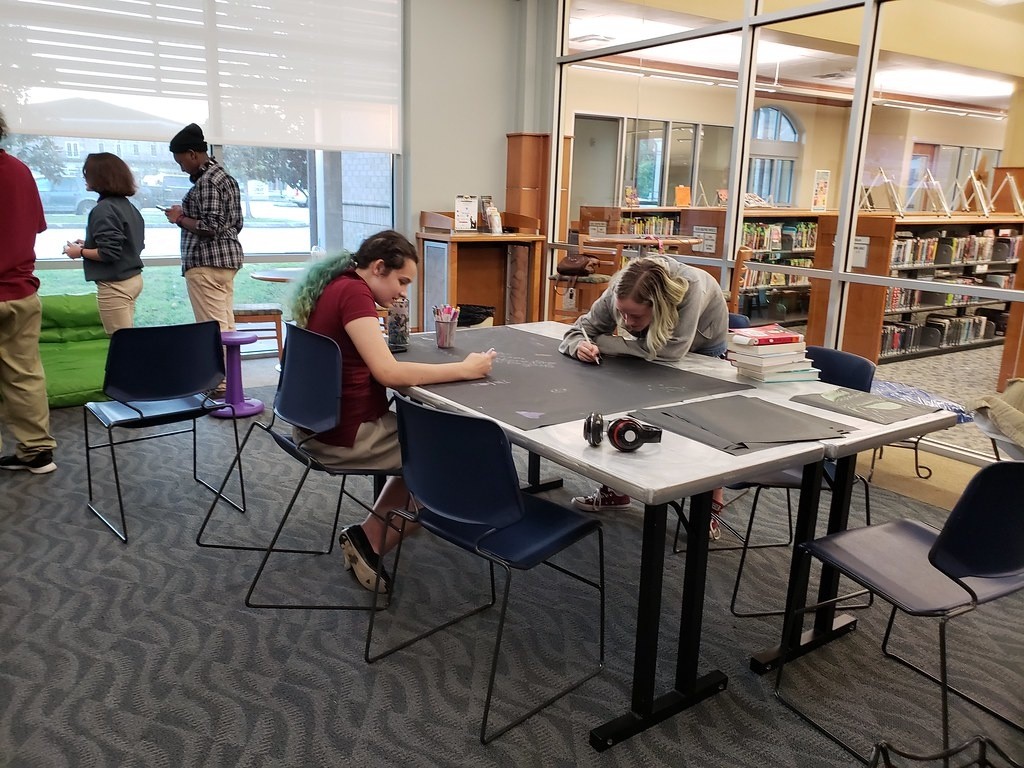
80 248 85 258
176 214 185 226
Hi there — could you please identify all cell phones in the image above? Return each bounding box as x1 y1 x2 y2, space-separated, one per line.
156 205 166 211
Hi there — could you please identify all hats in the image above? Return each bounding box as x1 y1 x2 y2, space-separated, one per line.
169 124 208 153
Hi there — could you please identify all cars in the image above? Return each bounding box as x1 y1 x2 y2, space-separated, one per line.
34 174 143 216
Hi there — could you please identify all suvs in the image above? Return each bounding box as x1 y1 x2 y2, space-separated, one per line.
286 179 308 208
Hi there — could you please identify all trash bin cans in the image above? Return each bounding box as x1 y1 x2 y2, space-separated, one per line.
457 304 493 330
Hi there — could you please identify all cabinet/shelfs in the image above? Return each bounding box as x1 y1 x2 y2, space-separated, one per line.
579 204 1023 392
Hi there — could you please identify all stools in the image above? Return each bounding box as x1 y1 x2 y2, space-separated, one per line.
207 332 264 417
376 305 389 334
230 304 283 361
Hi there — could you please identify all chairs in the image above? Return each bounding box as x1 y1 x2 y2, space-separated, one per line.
194 320 410 611
773 461 1024 767
673 345 876 618
83 321 246 543
549 235 625 324
721 245 752 314
364 390 605 745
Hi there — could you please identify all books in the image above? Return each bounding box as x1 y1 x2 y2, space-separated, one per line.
880 228 1022 352
625 186 639 207
729 322 823 384
739 221 818 288
620 217 674 263
675 186 690 206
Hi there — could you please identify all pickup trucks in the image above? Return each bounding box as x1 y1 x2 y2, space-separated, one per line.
133 175 195 208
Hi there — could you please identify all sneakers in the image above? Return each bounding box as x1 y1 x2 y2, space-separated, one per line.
571 484 632 511
0 451 57 473
710 497 725 537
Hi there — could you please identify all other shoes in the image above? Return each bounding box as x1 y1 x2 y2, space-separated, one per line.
340 525 392 593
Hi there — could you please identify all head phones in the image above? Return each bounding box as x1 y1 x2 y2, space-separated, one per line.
189 158 216 184
582 411 663 452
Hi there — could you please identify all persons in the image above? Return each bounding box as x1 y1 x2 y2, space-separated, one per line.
165 123 246 399
64 152 145 341
0 116 58 475
287 230 498 591
558 253 730 542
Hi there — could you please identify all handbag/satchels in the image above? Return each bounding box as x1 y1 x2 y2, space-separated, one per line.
554 254 600 295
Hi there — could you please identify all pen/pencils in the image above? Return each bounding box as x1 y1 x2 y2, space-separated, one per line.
486 348 493 353
432 304 460 347
578 321 601 365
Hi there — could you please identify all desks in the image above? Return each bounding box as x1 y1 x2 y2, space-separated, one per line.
972 412 1024 462
386 321 956 754
416 210 546 334
251 267 306 284
585 234 703 271
864 380 974 482
